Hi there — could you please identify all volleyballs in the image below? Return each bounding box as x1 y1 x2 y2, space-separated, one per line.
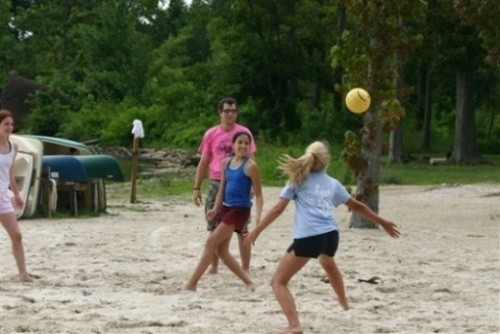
344 88 372 114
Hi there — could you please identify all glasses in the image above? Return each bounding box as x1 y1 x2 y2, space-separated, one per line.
222 109 237 114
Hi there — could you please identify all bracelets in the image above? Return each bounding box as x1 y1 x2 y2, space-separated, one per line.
194 187 200 190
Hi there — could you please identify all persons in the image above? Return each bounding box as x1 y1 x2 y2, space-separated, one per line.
184 132 265 293
242 139 401 334
0 109 35 282
192 97 257 276
